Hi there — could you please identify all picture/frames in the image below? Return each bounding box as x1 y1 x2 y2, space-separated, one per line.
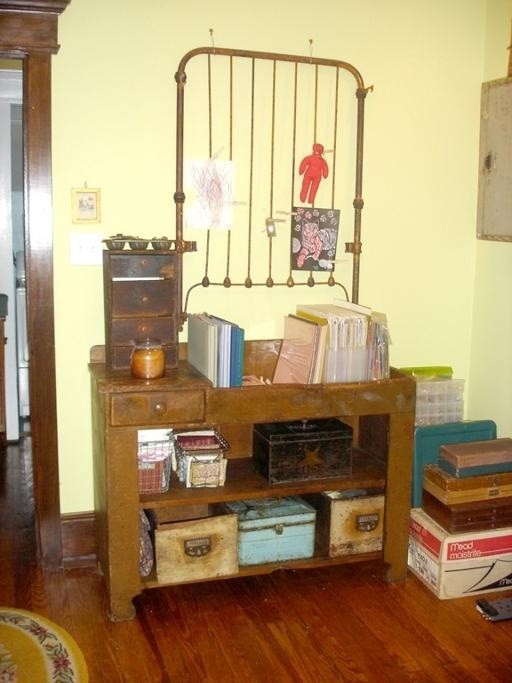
72 188 102 222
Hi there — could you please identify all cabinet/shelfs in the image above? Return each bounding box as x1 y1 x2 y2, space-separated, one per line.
104 249 179 371
88 344 415 621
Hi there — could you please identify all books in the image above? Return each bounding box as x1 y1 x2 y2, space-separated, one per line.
185 299 390 388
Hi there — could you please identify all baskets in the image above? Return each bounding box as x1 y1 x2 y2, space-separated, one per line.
414 379 463 426
137 440 172 494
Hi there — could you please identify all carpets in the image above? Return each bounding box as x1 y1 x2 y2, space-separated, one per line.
1 605 89 683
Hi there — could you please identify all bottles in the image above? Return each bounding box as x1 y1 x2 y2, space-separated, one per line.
129 336 167 380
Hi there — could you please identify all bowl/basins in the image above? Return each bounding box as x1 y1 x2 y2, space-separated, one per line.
100 239 172 249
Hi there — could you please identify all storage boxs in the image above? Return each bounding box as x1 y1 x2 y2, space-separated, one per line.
316 489 384 558
225 496 316 566
252 418 352 485
407 508 512 600
150 502 239 585
